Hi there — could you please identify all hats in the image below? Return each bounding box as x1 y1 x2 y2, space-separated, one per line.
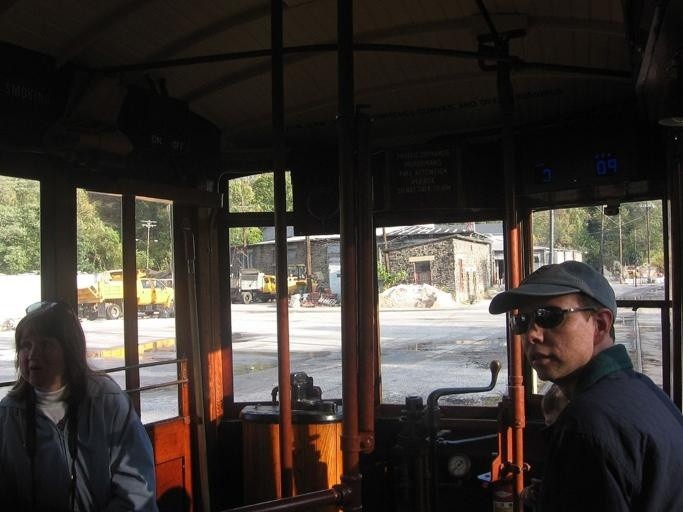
489 261 617 324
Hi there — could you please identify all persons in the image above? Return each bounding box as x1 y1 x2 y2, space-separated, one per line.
488 260 683 511
0 308 156 511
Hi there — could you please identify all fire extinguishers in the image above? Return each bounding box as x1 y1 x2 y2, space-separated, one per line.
491 452 515 512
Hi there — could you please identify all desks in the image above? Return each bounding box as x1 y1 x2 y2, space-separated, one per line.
240 404 345 495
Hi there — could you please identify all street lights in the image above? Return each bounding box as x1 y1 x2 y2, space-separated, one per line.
136 219 158 269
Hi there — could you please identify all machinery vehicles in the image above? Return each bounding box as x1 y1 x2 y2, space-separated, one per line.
287 263 325 293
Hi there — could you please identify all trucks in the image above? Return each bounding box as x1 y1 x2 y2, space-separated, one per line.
232 272 296 305
77 271 174 319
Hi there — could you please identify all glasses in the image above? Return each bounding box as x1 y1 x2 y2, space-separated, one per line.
509 306 597 335
26 301 80 319
